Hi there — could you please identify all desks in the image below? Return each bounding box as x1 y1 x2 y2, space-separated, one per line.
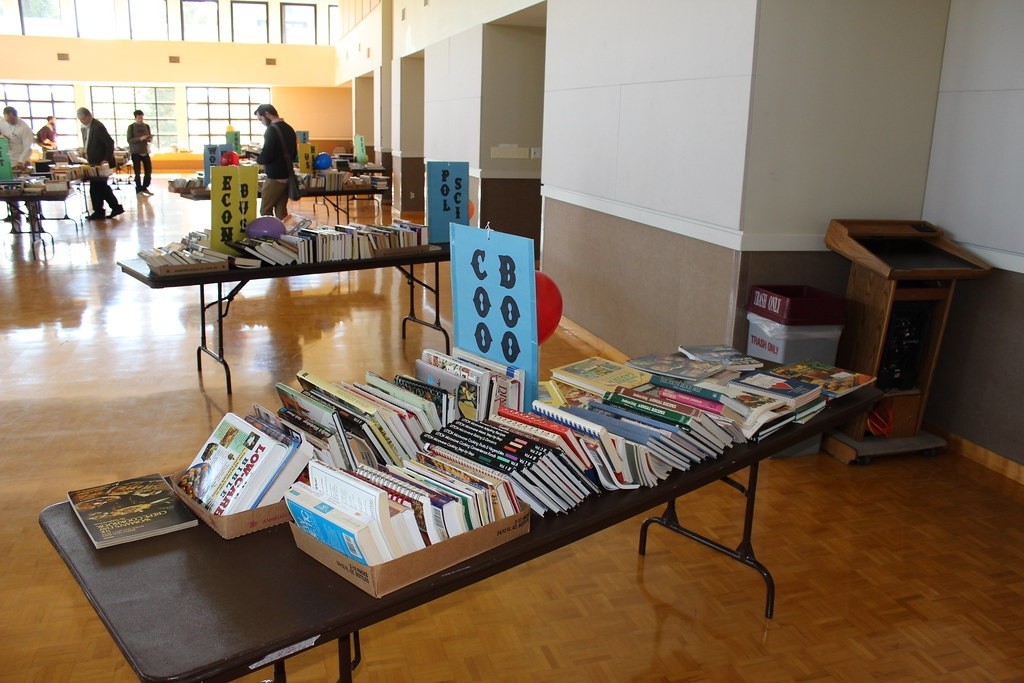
116 242 451 397
0 146 114 265
37 344 888 683
168 175 389 225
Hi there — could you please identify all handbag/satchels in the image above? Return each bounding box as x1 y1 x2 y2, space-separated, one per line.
288 173 301 200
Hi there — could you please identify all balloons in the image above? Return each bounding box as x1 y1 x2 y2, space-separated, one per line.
313 152 332 170
221 151 239 166
468 199 474 220
535 270 563 347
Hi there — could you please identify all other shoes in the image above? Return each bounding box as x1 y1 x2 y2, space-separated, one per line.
106 205 124 219
26 214 45 220
4 214 20 221
136 186 153 195
85 209 105 219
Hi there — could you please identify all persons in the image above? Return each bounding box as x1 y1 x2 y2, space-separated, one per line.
0 106 44 233
37 116 58 152
76 107 125 219
253 104 300 220
126 109 156 196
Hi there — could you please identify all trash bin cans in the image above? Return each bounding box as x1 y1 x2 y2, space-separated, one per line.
745 284 846 458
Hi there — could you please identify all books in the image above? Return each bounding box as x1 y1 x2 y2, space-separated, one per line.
136 212 429 278
167 170 391 195
67 341 876 600
0 147 130 196
326 153 385 169
